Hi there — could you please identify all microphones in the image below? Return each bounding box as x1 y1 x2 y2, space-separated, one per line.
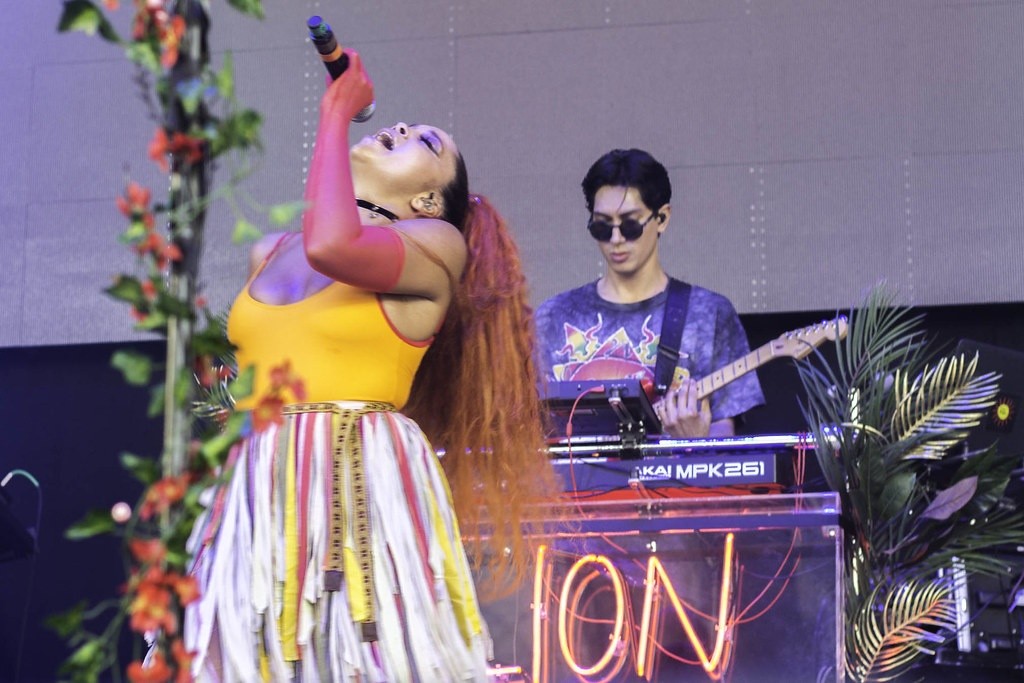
305 15 375 121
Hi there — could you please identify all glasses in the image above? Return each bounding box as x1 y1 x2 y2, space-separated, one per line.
587 208 656 242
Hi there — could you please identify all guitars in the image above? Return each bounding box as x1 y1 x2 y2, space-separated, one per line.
653 315 851 426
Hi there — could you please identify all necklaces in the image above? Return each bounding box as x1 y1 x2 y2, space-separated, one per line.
356 197 399 222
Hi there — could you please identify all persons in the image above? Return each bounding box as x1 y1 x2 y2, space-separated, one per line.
140 50 577 683
532 148 765 440
